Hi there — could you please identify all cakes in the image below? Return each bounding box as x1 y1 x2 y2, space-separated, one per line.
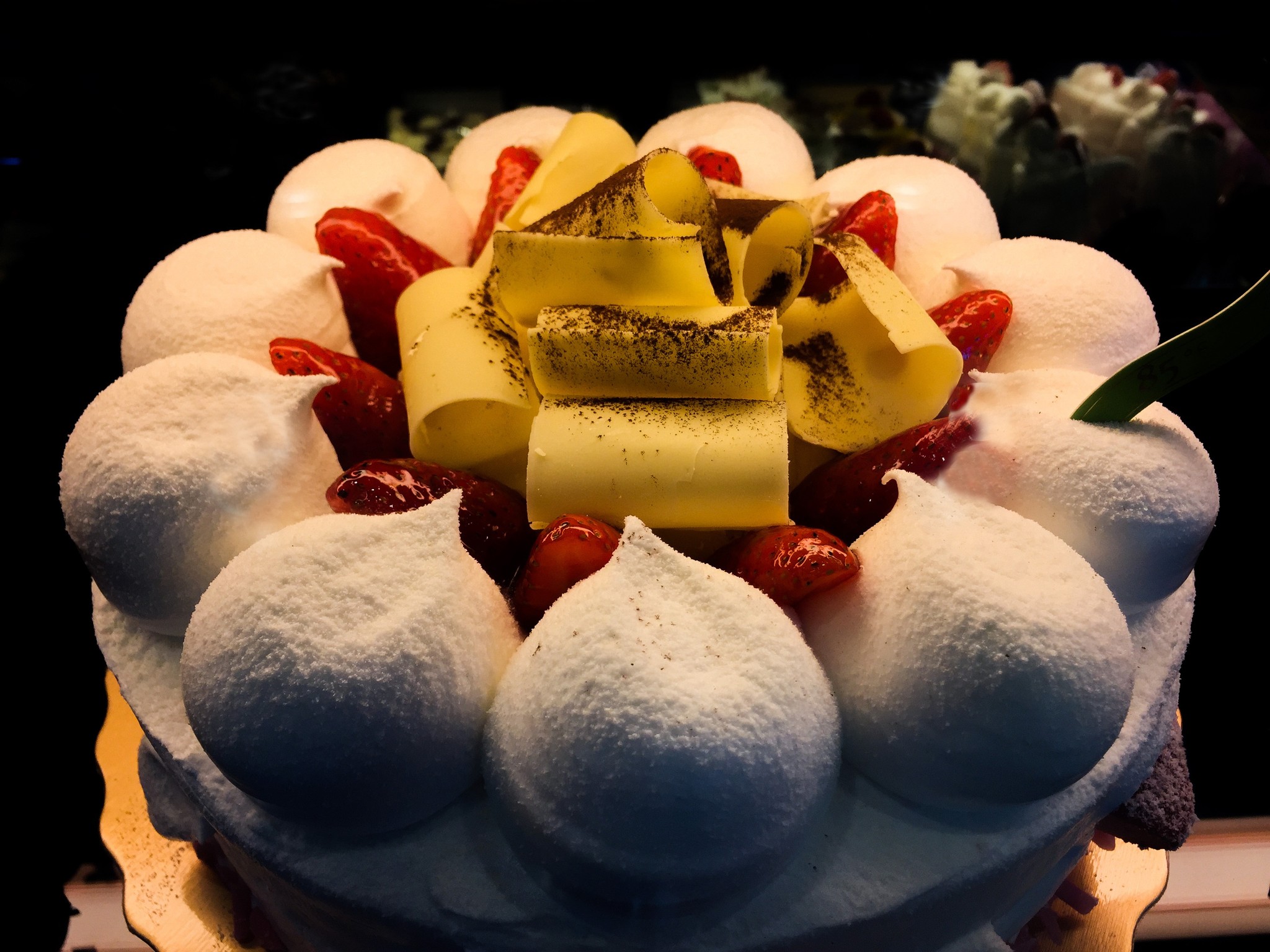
59 99 1222 952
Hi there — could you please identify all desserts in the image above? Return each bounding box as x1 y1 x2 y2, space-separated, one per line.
927 58 1236 207
